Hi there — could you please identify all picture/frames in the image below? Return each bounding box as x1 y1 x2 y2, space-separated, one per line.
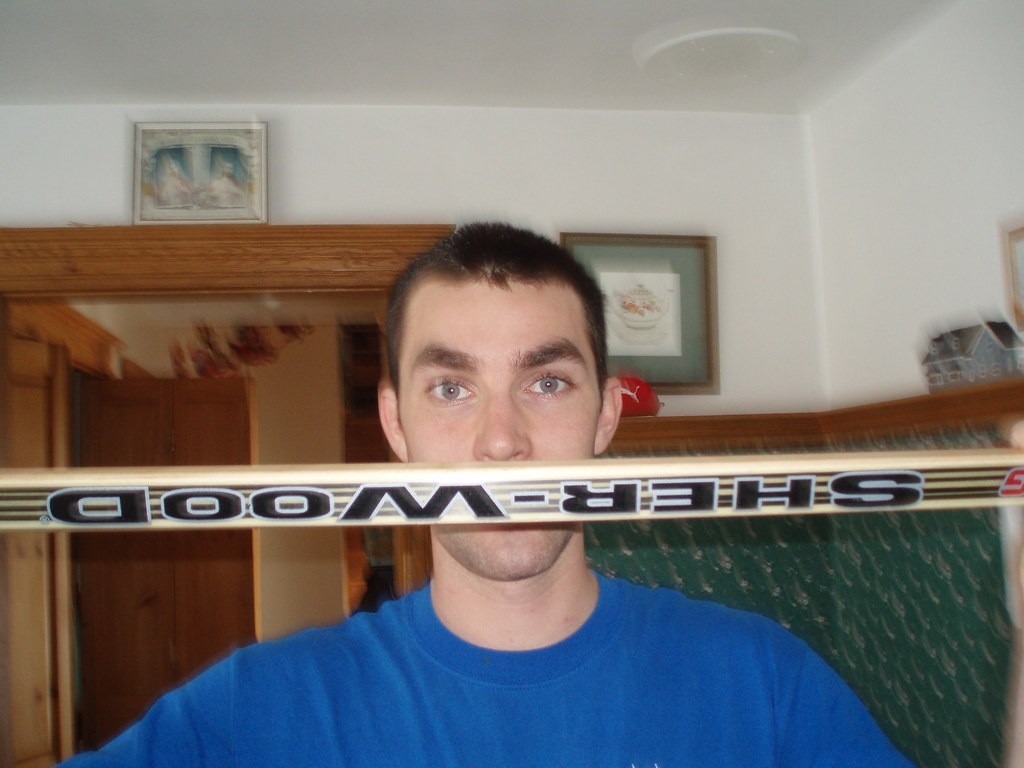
560 231 721 396
133 119 268 223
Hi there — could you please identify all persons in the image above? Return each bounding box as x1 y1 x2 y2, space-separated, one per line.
49 222 1024 768
